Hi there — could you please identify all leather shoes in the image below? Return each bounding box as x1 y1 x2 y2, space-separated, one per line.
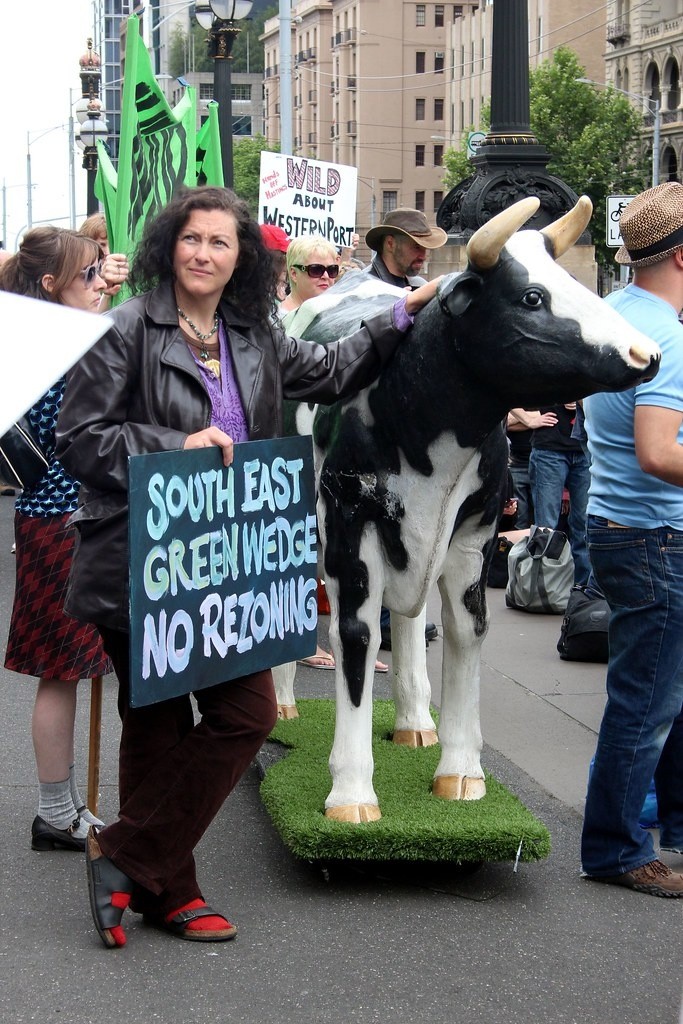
381 624 429 647
426 622 438 639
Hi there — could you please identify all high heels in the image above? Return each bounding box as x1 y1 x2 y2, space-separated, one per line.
76 805 109 831
31 814 86 852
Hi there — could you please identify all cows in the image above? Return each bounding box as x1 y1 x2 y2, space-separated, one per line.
283 196 662 823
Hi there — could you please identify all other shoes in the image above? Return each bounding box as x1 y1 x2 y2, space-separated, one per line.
660 845 683 854
584 859 683 898
0 486 16 497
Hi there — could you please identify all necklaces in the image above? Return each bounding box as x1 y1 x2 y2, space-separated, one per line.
175 306 219 362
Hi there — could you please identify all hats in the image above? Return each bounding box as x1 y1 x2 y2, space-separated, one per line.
365 207 448 252
258 224 291 253
614 182 683 269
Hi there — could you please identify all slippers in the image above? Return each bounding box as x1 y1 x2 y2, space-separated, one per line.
85 825 133 948
374 659 388 672
297 654 335 669
143 895 237 940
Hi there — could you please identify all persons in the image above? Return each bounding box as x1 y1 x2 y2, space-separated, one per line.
56 188 445 949
0 225 129 851
259 206 593 591
580 181 683 899
0 211 109 495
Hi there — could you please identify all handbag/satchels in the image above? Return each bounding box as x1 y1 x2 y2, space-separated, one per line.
557 583 612 663
505 525 575 614
487 537 514 589
0 416 49 492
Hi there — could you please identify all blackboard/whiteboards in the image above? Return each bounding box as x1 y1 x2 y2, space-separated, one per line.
257 150 358 249
128 435 323 710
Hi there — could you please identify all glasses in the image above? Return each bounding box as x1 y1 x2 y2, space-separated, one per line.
35 259 103 289
290 263 339 278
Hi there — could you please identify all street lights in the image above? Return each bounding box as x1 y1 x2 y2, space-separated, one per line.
575 78 661 188
73 37 108 220
195 0 254 189
